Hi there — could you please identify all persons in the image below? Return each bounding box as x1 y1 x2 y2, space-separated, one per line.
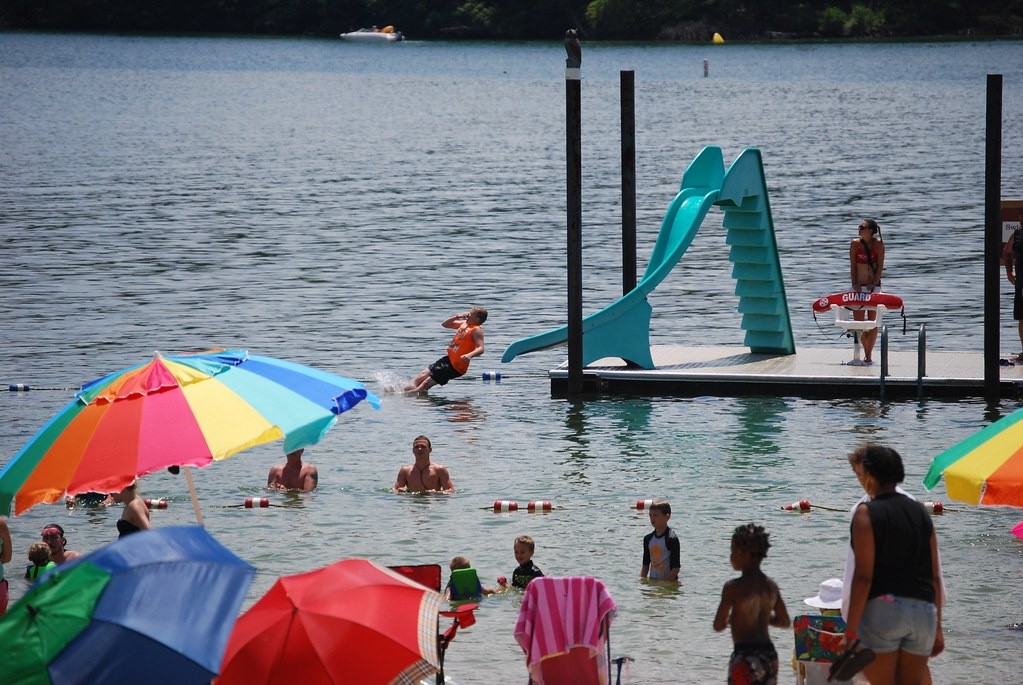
0 516 13 619
850 219 885 363
714 523 791 685
24 523 81 585
444 557 505 603
408 307 489 396
394 435 456 493
511 535 545 590
112 478 152 541
639 500 680 583
267 447 317 493
792 576 846 677
1003 206 1023 364
835 444 945 685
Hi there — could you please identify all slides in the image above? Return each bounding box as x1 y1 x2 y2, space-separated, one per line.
501 186 723 364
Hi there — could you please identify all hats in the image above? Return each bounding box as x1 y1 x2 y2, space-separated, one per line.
804 579 843 609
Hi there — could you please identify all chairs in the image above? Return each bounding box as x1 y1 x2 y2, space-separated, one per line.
829 286 886 366
519 578 634 685
384 564 480 685
793 613 848 684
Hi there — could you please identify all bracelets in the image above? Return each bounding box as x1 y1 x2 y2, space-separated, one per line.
844 630 859 639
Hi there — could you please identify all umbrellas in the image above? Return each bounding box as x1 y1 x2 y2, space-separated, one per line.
925 403 1023 511
0 346 368 527
208 557 444 685
1 525 257 685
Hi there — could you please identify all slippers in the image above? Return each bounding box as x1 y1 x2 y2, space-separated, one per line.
827 639 875 683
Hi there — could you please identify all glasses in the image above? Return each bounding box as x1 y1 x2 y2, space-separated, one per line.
858 224 866 230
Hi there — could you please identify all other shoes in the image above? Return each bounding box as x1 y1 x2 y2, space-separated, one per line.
1000 359 1015 367
